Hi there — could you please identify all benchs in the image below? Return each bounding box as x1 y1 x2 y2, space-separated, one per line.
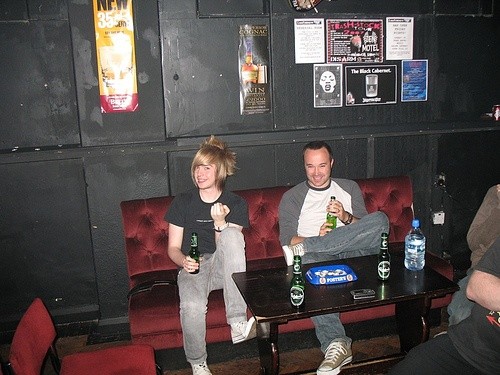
120 177 454 351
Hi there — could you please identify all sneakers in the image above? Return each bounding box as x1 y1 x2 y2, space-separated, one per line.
282 242 304 266
192 361 212 375
231 316 257 344
317 341 352 375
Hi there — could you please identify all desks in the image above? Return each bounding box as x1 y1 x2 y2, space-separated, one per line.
231 252 460 375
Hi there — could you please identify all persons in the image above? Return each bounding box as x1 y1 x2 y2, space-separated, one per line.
448 184 500 326
163 136 266 375
387 235 500 375
278 142 390 375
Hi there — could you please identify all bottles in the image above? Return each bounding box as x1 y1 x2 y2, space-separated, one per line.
289 255 305 311
325 196 337 233
376 233 391 285
188 232 200 274
404 219 426 272
241 36 259 84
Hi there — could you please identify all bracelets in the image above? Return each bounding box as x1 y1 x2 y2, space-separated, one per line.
214 222 229 232
341 212 353 225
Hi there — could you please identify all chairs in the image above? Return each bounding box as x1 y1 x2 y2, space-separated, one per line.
7 298 162 375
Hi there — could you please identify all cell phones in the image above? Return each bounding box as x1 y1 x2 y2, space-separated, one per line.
350 289 376 299
